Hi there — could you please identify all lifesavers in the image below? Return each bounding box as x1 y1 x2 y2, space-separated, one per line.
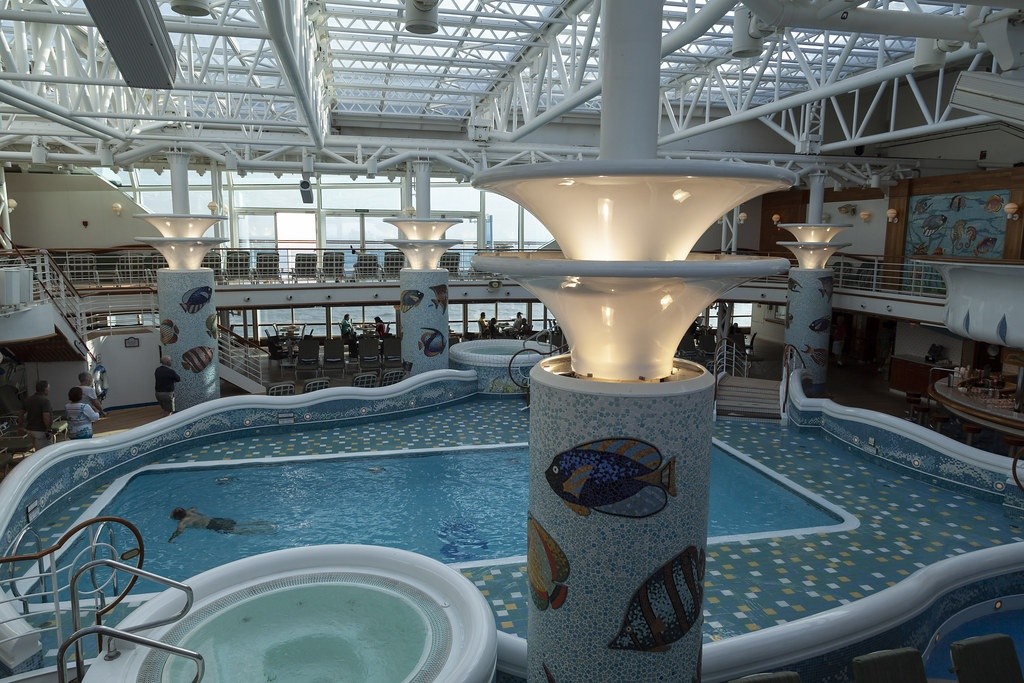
94 365 108 397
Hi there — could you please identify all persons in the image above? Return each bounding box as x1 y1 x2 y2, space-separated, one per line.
167 505 279 544
63 386 100 439
75 371 109 428
478 311 490 339
340 313 360 341
516 318 532 340
727 320 742 336
372 315 385 340
488 318 504 339
153 355 181 418
512 311 524 331
15 379 54 452
